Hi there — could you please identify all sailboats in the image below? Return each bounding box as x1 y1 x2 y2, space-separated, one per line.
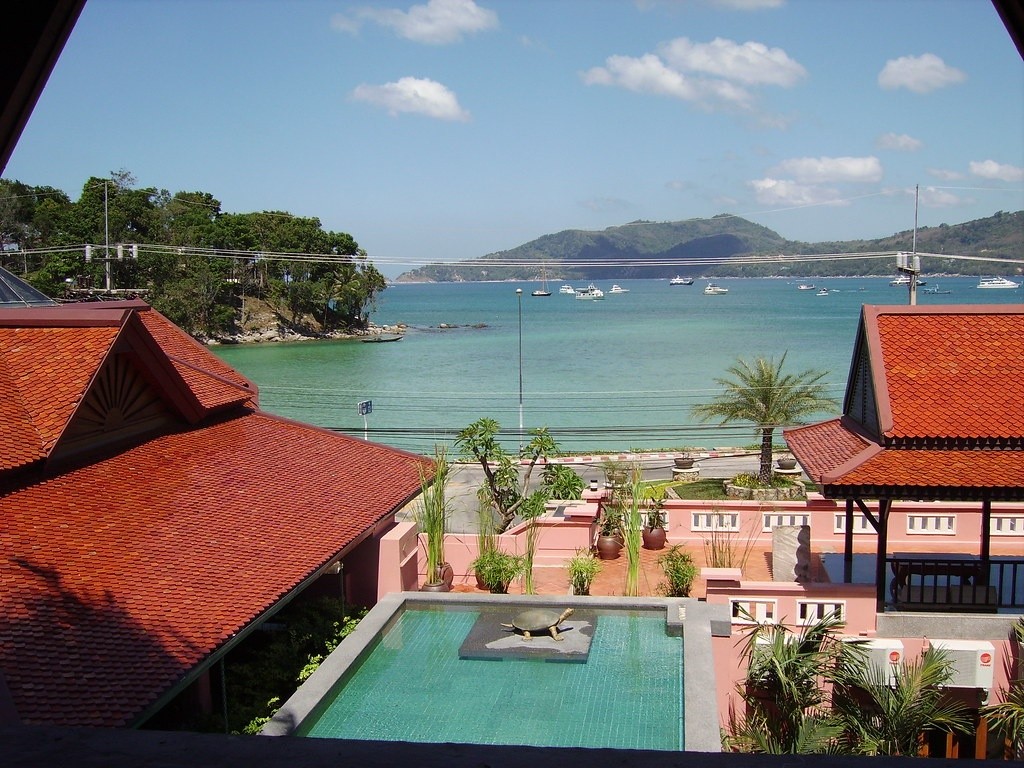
530 258 554 296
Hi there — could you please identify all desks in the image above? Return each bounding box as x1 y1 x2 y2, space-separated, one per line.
889 552 979 602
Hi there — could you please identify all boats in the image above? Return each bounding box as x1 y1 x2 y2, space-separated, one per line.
797 282 817 290
559 281 605 301
976 274 1021 289
608 284 631 295
815 288 830 296
669 274 695 286
702 282 729 296
888 275 927 287
360 335 404 343
921 283 951 295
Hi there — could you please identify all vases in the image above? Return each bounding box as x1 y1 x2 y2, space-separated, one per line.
777 459 798 469
675 458 694 468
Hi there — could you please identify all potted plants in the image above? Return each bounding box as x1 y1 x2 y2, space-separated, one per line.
729 598 847 741
403 439 666 594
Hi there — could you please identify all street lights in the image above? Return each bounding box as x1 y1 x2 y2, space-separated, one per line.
515 288 523 461
